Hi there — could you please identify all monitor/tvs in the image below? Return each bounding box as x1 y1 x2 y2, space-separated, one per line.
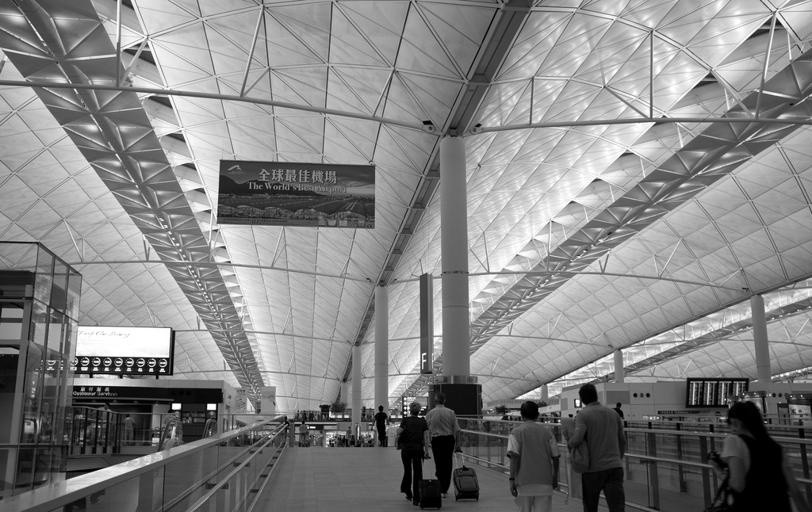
434 383 482 417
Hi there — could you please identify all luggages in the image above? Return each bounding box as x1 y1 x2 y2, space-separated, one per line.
416 454 443 510
453 447 480 501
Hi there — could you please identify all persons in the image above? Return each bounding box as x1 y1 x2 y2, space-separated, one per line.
370 406 390 447
395 402 431 506
328 426 376 447
27 411 55 467
425 392 463 501
502 412 522 435
361 406 376 422
711 401 810 511
567 383 627 512
97 401 113 421
294 409 324 448
613 402 625 422
539 409 580 466
122 414 136 446
506 401 561 512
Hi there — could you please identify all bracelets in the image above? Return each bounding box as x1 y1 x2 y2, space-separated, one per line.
509 477 515 481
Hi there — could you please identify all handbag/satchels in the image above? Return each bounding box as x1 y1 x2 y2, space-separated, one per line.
700 475 730 511
